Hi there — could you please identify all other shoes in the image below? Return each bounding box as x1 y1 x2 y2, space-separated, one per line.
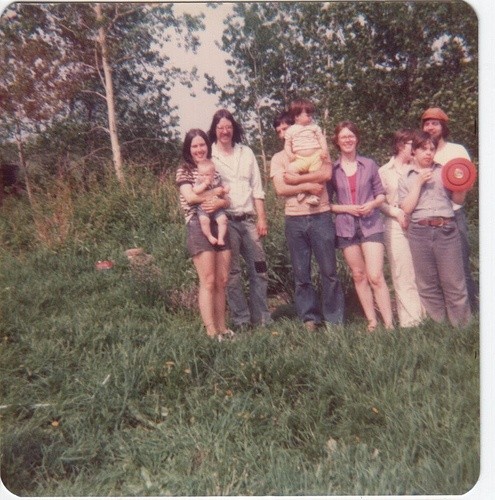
306 320 316 331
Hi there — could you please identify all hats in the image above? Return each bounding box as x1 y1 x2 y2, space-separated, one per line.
421 108 448 122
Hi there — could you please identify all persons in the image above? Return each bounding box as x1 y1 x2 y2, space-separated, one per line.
411 108 477 314
179 108 277 331
192 160 228 246
326 122 396 333
398 132 479 330
174 129 240 341
284 99 329 207
374 128 434 329
269 112 348 333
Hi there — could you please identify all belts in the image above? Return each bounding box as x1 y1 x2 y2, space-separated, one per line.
417 218 452 227
225 213 251 221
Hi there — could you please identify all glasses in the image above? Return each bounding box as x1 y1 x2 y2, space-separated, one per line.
215 125 232 131
